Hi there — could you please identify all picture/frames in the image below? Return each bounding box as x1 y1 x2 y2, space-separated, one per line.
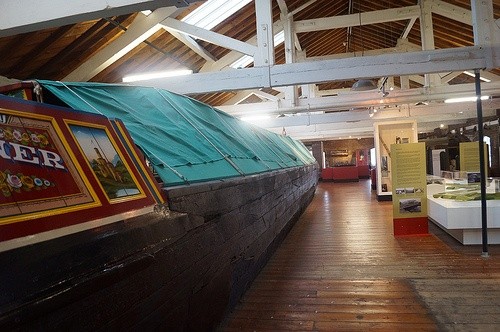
0 94 170 254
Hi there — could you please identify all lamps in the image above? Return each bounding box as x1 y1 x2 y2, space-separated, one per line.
351 0 378 92
